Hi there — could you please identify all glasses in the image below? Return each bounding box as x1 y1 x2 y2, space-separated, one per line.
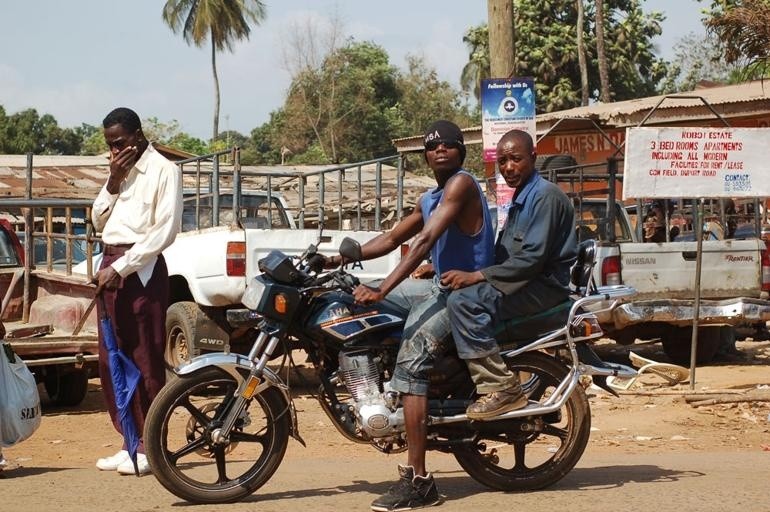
425 142 460 149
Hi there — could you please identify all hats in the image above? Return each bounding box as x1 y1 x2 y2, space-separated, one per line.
421 120 465 146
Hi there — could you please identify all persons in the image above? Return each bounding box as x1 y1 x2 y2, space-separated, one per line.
702 196 739 241
634 194 681 243
0 299 7 472
87 106 185 478
413 127 584 422
305 118 498 512
669 209 686 228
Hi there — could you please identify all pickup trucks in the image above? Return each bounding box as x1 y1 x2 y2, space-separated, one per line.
469 95 770 370
66 146 430 399
0 194 100 411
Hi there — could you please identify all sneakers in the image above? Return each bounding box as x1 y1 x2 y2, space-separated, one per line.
97 451 130 469
370 464 440 511
117 453 152 475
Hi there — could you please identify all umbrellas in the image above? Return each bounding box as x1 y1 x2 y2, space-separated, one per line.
90 277 142 477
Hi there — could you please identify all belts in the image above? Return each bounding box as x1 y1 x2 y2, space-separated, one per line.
100 244 132 255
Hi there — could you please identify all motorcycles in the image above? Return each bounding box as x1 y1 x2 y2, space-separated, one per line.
135 223 638 502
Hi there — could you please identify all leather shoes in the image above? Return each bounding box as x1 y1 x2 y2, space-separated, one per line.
467 384 528 418
